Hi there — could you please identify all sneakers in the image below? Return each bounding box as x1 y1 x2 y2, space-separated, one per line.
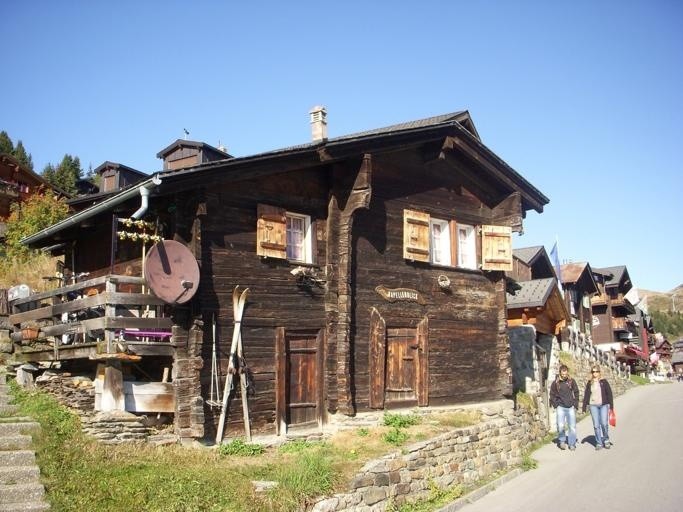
595 440 610 450
557 441 575 451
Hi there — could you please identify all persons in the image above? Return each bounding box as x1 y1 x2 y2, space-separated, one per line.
580 365 615 451
547 365 580 451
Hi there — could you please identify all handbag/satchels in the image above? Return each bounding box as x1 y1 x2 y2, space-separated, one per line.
609 411 616 426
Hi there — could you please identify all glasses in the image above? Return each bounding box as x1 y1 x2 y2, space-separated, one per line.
592 371 599 373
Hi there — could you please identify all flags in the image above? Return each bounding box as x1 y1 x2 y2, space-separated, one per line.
549 240 560 281
